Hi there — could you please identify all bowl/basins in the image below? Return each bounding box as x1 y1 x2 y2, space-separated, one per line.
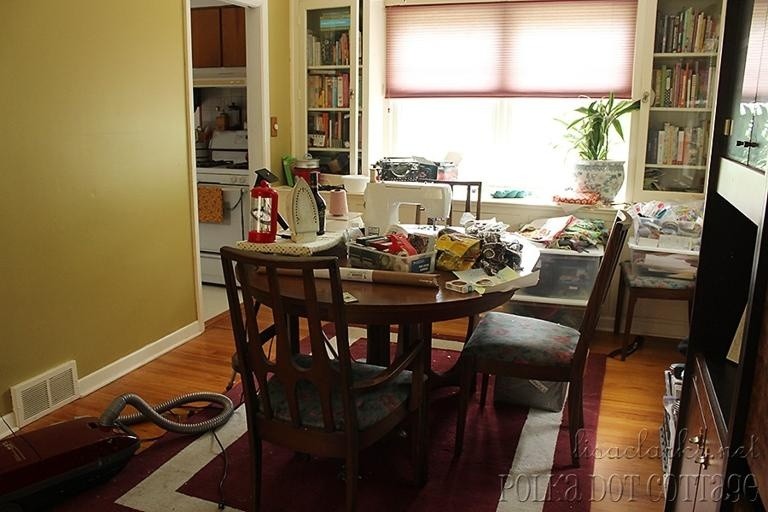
341 175 368 193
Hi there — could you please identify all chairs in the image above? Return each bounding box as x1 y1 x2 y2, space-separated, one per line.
213 176 632 511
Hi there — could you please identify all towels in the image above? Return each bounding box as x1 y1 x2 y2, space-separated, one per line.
198 185 224 225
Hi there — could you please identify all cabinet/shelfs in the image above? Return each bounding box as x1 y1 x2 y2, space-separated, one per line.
190 1 246 80
289 0 387 192
630 1 768 510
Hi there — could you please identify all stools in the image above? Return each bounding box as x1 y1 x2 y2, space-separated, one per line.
611 259 696 362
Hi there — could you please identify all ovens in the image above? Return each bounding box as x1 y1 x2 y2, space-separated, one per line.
197 173 250 260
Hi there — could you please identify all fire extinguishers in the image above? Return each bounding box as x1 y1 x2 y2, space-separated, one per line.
248 168 289 243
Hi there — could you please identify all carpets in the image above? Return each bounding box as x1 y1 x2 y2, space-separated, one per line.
64 323 607 511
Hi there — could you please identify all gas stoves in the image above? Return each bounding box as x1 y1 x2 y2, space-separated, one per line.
197 159 248 170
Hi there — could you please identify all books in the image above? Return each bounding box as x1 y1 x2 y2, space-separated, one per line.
640 3 720 190
307 27 363 178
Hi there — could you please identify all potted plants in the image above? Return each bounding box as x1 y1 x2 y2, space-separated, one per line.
545 91 642 206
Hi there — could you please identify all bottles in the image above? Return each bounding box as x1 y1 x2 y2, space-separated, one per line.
227 101 244 131
309 171 326 236
247 180 278 243
